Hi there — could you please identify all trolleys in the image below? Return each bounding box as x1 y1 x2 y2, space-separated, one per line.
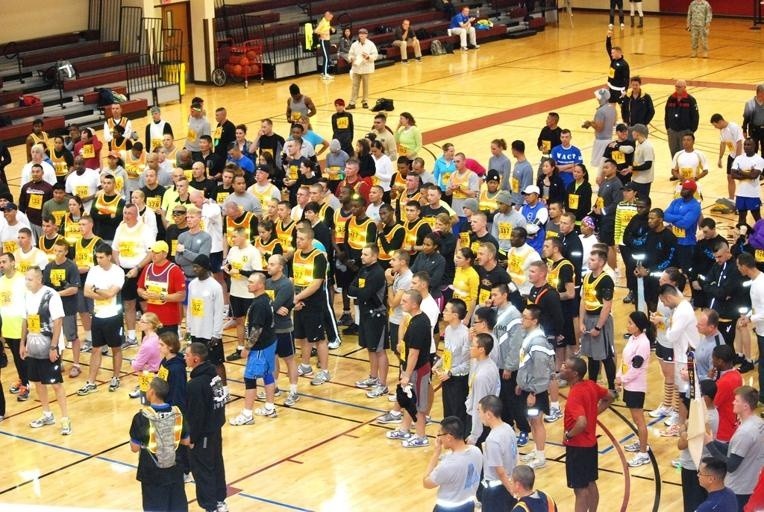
211 37 268 89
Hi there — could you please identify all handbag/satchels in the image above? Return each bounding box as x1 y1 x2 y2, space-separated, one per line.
373 22 393 35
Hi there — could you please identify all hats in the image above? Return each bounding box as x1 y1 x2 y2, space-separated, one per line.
462 168 541 213
149 240 169 254
1 202 17 212
107 150 121 159
679 179 697 193
594 88 611 100
616 124 648 137
619 181 640 192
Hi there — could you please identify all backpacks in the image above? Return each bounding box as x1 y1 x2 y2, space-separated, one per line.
430 39 454 55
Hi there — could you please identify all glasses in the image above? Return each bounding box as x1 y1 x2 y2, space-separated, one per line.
437 432 453 437
140 320 150 324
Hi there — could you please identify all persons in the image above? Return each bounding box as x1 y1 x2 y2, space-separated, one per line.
344 28 378 110
609 29 625 50
608 1 625 27
391 19 422 65
628 1 644 28
1 75 764 511
338 29 353 65
686 0 713 59
434 1 456 21
314 11 335 80
630 28 644 55
604 29 628 123
448 7 479 50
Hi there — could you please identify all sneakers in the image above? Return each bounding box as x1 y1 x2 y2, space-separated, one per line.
69 335 142 398
689 48 709 58
732 353 754 374
625 402 682 468
608 22 625 29
460 44 480 51
514 371 566 469
401 56 423 63
320 72 335 80
345 103 369 110
226 314 359 426
206 499 230 512
354 376 430 447
9 363 71 436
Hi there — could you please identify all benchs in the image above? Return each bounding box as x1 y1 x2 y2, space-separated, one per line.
1 26 180 155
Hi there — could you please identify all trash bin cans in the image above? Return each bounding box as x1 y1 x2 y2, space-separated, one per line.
161 60 186 96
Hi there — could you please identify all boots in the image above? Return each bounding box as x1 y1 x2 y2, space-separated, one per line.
630 15 644 28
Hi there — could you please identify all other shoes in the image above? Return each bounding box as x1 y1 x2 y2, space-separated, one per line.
182 472 193 482
